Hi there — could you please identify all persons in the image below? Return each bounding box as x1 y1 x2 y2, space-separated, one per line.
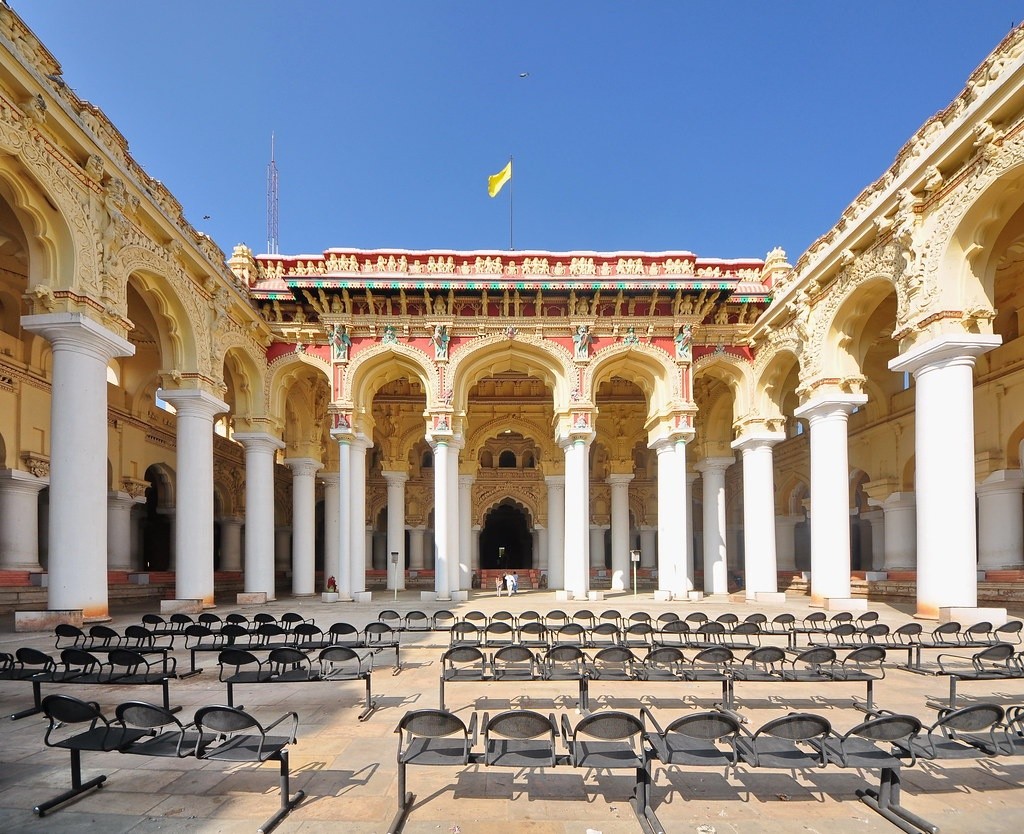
327 576 337 593
496 571 518 597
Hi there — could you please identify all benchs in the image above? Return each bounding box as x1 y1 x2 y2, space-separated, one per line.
0 610 1024 834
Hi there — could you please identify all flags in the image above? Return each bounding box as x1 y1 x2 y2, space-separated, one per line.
488 161 511 197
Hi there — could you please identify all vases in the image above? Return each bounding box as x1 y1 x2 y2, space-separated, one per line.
128 573 150 586
866 572 888 582
